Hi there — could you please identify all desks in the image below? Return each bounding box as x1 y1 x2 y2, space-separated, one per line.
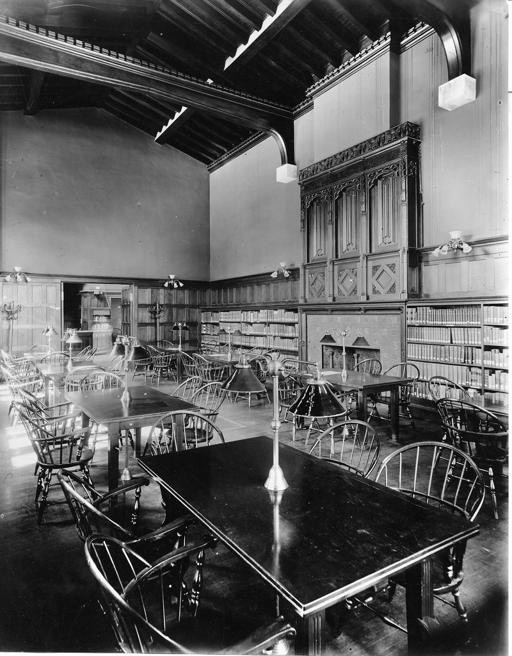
136 436 479 584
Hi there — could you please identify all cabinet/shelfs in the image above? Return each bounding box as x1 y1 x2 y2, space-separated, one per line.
201 306 299 369
406 301 508 416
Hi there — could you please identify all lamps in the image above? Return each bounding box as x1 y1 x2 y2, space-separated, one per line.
432 231 473 257
271 263 291 278
164 274 184 289
41 320 369 400
5 267 31 283
222 342 347 492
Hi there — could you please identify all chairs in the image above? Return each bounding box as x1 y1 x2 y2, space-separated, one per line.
376 441 486 655
57 469 197 620
87 534 297 653
